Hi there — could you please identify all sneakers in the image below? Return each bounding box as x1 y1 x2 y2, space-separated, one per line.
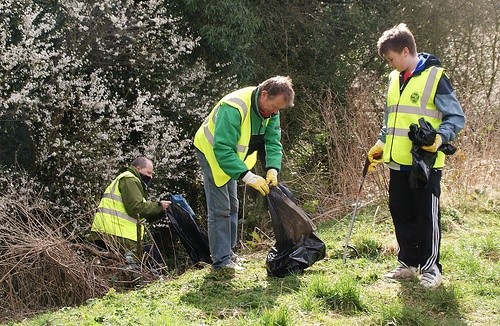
383 266 416 281
419 266 442 290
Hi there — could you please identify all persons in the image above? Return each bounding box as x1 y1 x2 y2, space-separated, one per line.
193 78 294 270
369 24 466 290
88 159 171 260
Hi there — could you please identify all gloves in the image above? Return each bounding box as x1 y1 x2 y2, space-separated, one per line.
367 140 384 166
243 171 270 196
266 168 278 186
421 134 442 152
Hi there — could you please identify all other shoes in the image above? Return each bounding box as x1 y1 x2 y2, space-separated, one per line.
221 262 245 271
